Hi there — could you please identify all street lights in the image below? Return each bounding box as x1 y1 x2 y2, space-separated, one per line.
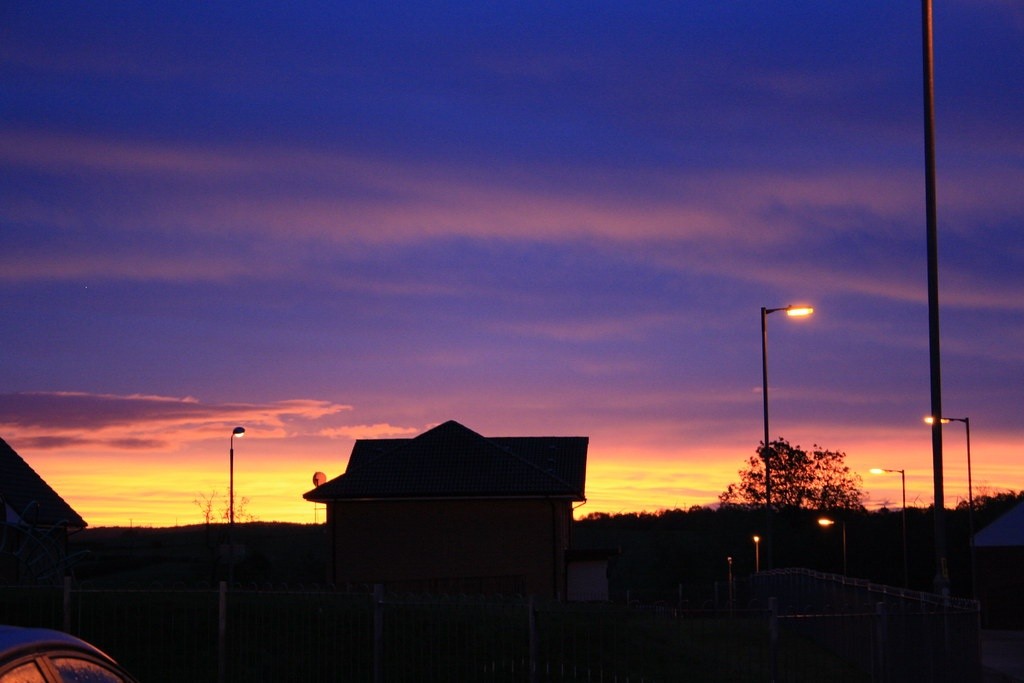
922 417 977 600
753 533 761 572
817 517 848 575
229 427 245 523
870 467 908 587
762 303 815 505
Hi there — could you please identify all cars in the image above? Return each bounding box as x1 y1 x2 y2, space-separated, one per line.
0 624 141 683
